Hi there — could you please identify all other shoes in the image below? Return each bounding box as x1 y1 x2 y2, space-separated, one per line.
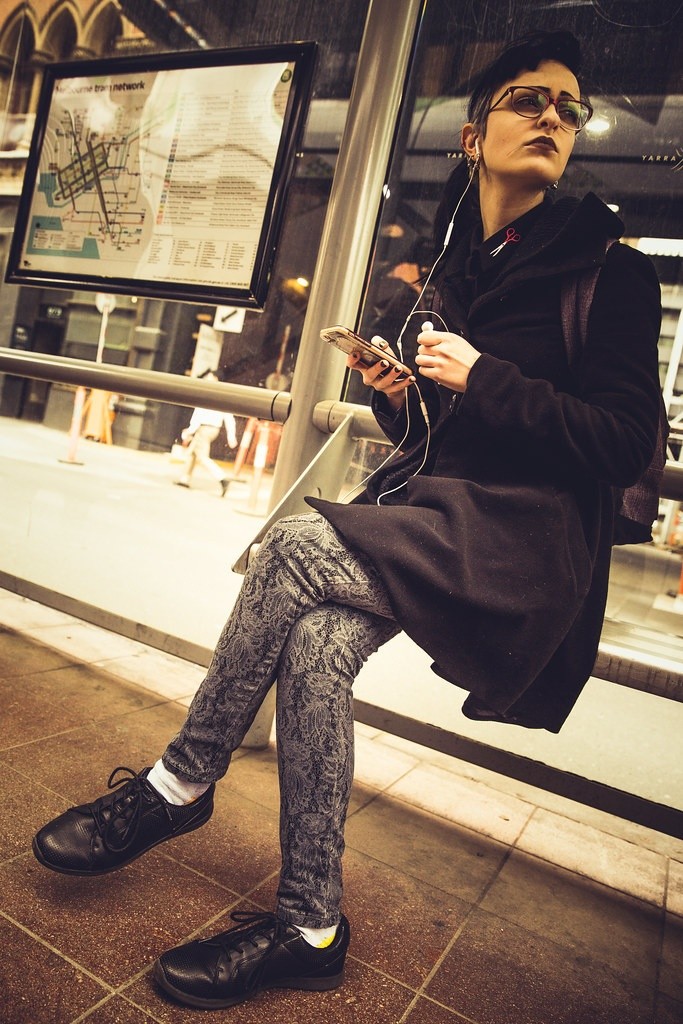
221 479 229 496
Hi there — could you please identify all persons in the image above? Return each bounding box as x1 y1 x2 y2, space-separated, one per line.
31 39 670 1010
173 367 239 497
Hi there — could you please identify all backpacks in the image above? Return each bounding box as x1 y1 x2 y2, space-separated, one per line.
561 235 669 548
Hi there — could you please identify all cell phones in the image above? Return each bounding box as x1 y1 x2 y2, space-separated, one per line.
319 324 413 382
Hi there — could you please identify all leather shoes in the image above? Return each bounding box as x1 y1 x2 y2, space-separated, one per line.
155 909 350 1011
33 767 213 877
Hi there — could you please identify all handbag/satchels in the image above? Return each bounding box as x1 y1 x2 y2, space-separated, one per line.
168 441 195 483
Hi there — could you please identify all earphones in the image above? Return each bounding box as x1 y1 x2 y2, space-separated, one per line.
421 320 434 332
475 137 480 156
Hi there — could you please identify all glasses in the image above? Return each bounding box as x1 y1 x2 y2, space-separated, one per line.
489 86 594 135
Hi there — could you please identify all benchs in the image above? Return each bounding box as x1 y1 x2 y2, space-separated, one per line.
231 411 683 704
